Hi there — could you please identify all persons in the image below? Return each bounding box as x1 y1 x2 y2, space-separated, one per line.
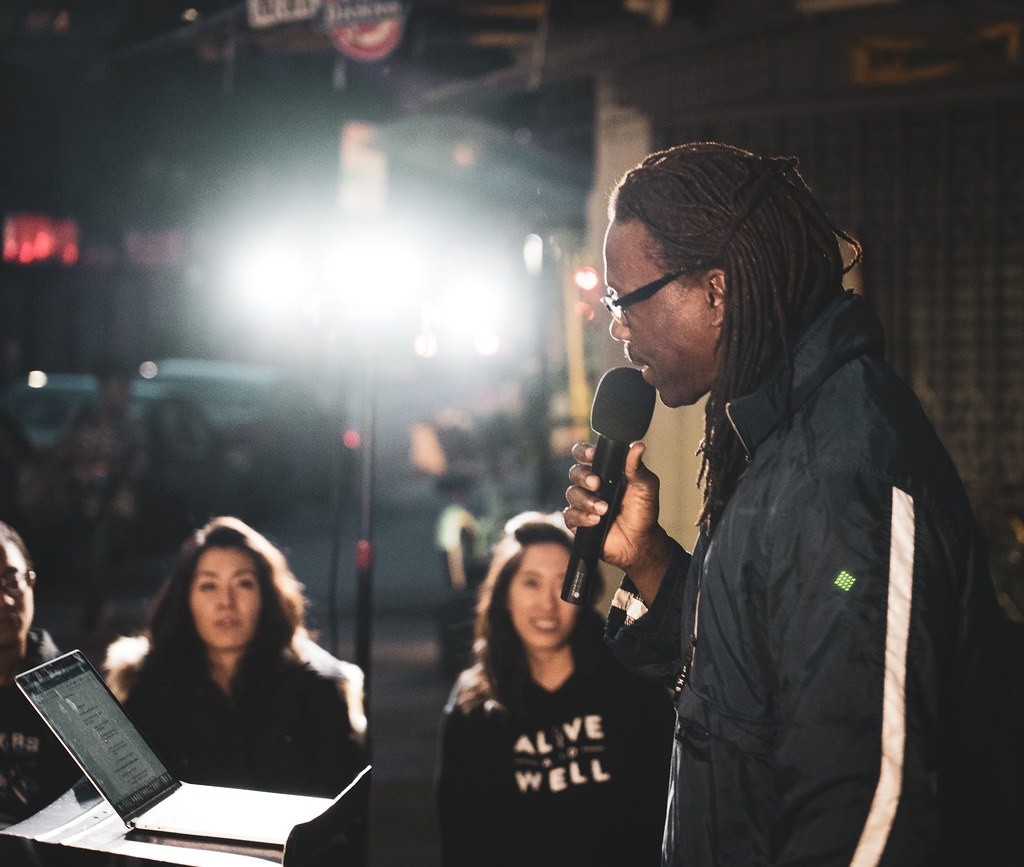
100 513 369 867
0 522 91 867
564 142 1024 867
435 518 675 867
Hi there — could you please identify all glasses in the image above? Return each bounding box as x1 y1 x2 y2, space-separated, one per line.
600 268 689 328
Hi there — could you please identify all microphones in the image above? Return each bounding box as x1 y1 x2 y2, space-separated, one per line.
561 368 656 606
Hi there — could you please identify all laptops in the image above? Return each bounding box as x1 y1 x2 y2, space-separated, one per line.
15 650 335 847
